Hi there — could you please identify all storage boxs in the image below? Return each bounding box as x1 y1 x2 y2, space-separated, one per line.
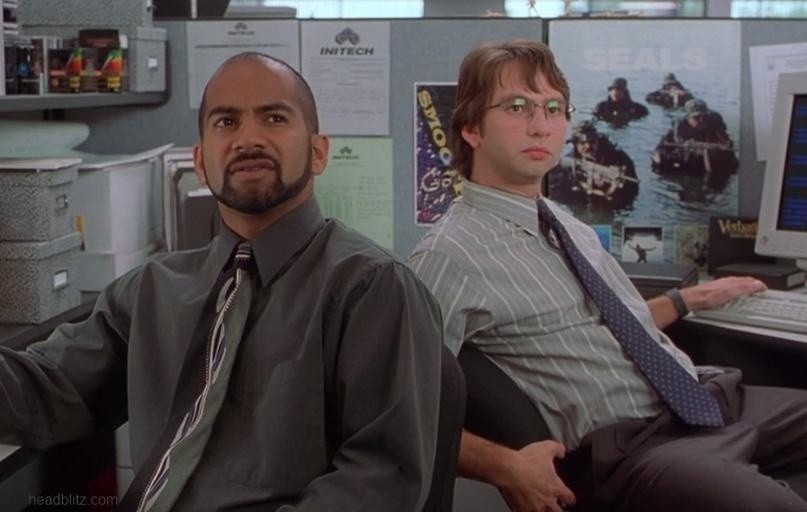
14 0 167 93
1 159 154 325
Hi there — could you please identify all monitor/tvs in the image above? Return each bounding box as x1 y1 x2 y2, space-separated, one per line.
751 70 807 271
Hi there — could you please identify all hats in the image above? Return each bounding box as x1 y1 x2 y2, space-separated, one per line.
684 99 711 117
608 79 626 90
565 121 602 143
663 74 680 88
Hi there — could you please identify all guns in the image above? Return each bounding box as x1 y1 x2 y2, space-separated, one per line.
663 138 737 172
579 109 629 120
561 156 639 194
661 90 687 108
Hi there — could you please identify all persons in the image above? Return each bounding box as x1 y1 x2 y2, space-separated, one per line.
403 38 807 512
593 78 648 125
651 98 733 174
0 50 441 512
550 122 640 209
646 72 695 109
625 242 659 262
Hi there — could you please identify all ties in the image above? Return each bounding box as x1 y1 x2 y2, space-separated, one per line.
120 241 253 512
535 198 725 430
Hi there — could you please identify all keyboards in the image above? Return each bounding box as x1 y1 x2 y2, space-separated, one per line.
695 287 807 333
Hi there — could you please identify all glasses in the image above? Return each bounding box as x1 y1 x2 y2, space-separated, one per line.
484 97 576 119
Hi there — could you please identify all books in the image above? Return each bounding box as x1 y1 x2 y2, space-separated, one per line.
714 263 807 291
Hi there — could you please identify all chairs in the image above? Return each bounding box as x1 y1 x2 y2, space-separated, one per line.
426 330 569 511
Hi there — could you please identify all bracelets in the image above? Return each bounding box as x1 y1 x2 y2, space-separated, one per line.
664 288 689 317
